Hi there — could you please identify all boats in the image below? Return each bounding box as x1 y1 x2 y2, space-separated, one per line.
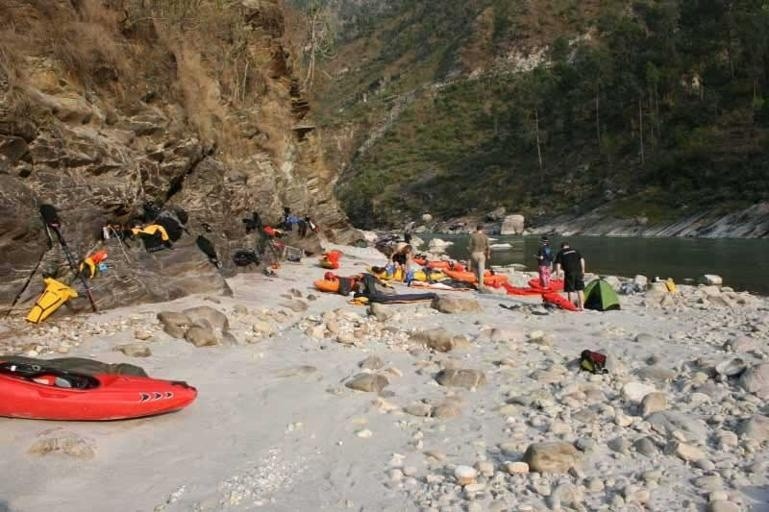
314 250 508 292
1 355 198 422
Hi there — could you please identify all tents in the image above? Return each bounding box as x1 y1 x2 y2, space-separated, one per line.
574 273 621 311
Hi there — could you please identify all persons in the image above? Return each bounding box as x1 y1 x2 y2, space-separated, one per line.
404 228 411 244
553 241 585 311
532 235 554 289
466 222 491 291
392 244 415 283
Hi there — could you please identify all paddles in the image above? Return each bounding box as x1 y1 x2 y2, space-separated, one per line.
423 267 435 284
41 204 100 312
253 211 280 267
